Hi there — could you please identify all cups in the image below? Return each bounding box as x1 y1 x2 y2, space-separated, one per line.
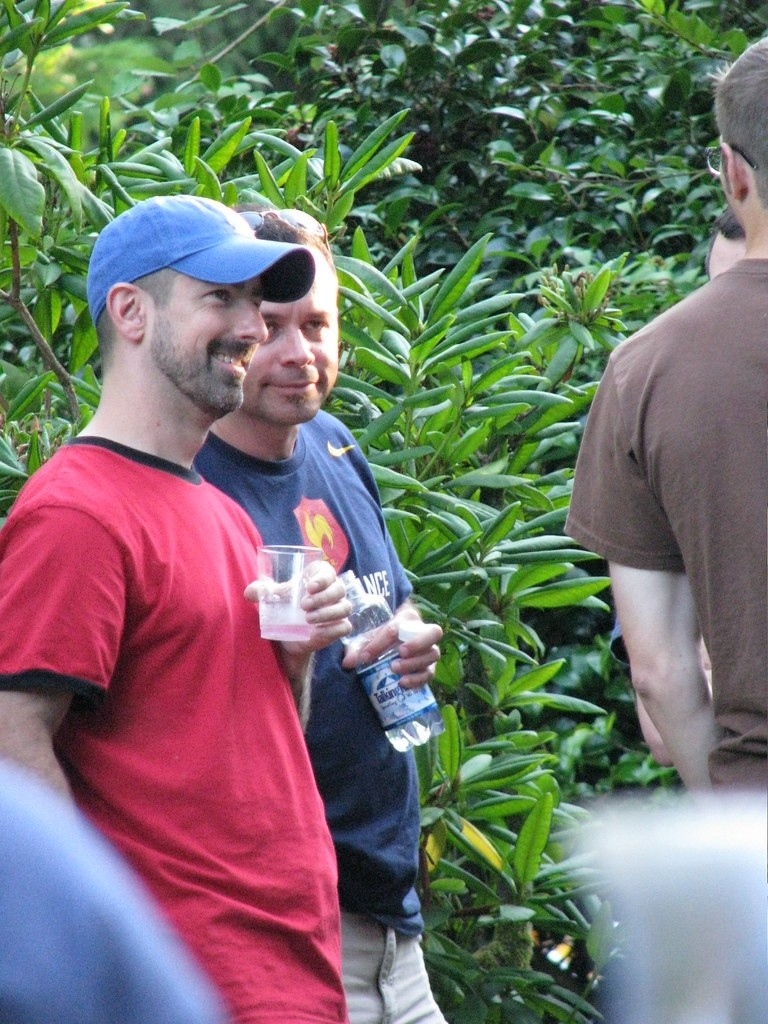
257 544 324 642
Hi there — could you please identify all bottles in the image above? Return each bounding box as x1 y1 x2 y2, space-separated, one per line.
337 570 446 753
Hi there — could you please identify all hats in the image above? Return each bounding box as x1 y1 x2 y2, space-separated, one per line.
86 194 316 324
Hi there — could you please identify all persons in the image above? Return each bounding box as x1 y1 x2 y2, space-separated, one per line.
200 209 445 1024
564 40 768 792
0 196 354 1024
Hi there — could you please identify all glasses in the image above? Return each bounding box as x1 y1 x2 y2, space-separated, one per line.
705 143 759 177
232 208 332 252
